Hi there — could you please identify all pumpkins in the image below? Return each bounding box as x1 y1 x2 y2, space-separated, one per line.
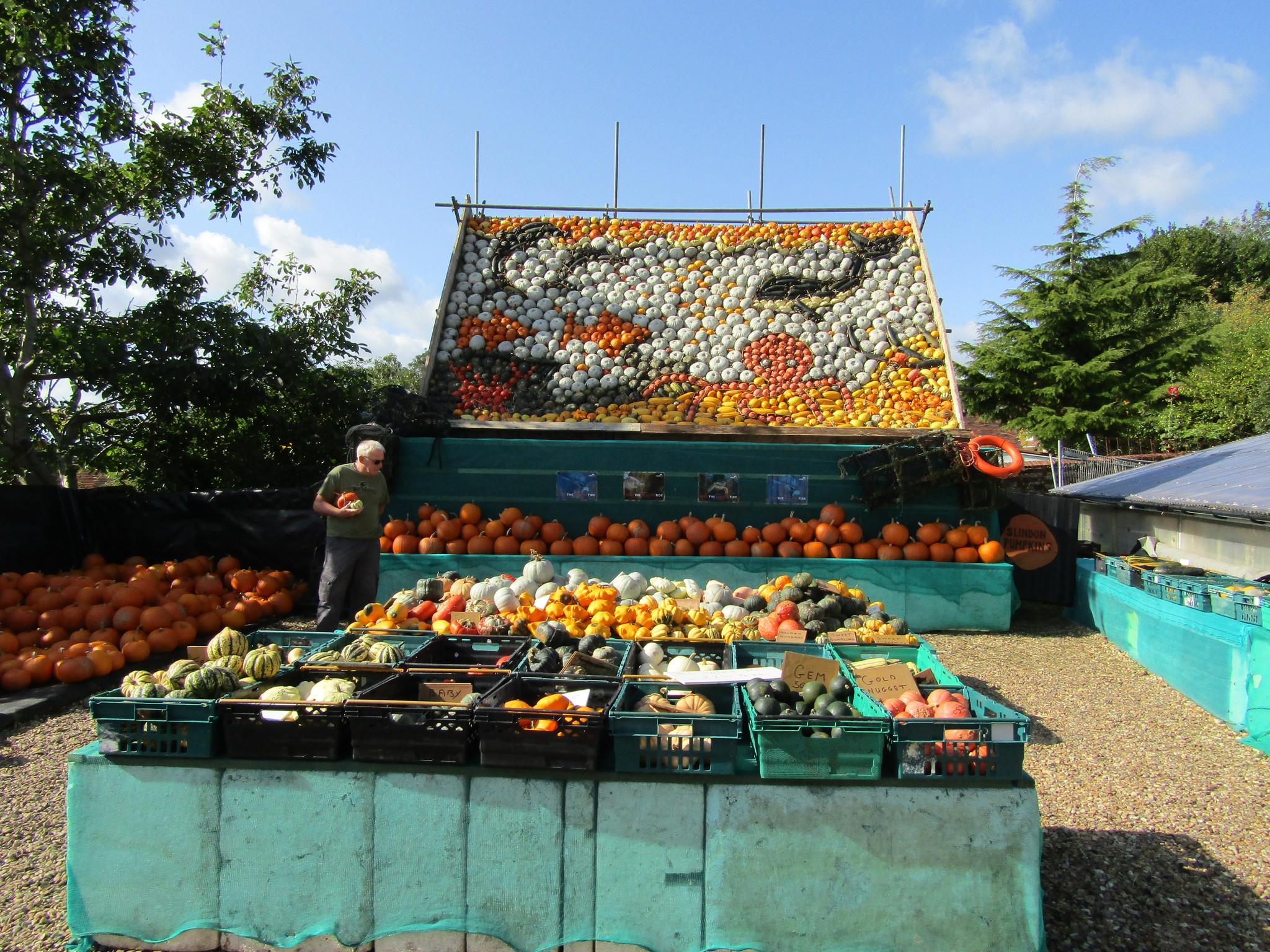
1123 555 1270 604
429 213 958 431
0 503 1005 769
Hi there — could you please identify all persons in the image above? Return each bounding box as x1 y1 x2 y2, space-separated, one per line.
313 438 396 634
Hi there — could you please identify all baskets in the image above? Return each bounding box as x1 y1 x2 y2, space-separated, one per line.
87 630 1034 784
1077 539 1270 631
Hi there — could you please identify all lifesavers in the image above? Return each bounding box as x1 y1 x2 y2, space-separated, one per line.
967 434 1025 479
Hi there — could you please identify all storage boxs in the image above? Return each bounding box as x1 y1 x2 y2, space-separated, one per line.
86 627 1034 784
1070 539 1102 558
1092 549 1269 630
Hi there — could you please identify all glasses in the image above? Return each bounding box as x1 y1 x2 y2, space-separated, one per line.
363 455 385 464
